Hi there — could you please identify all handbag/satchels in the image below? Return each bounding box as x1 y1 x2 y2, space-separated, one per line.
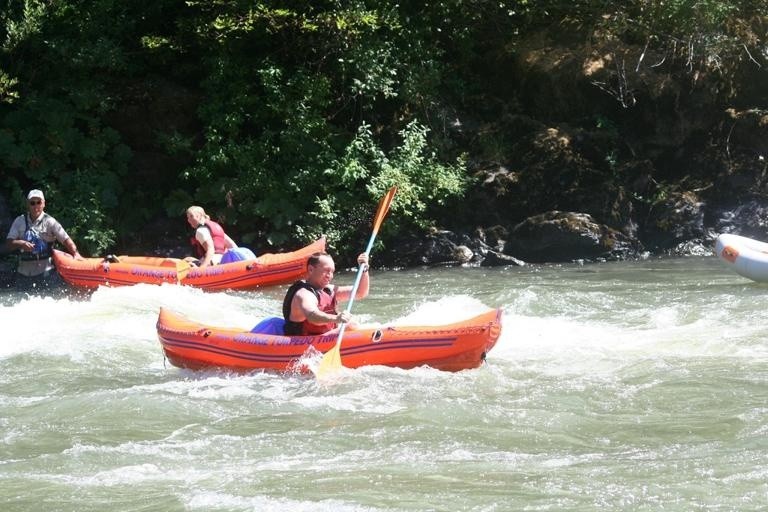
73 247 80 254
362 264 371 272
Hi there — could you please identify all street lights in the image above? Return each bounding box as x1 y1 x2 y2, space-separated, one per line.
23 212 48 254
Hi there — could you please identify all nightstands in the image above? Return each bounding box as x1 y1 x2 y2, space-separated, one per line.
318 186 397 366
176 260 194 280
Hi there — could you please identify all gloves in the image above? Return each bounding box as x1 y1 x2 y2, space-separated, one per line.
158 306 502 378
49 236 326 293
714 233 767 280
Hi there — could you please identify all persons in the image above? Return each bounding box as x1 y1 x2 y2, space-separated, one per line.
3 187 86 293
185 205 241 270
279 251 372 335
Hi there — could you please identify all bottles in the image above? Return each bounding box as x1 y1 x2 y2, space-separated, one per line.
27 189 44 201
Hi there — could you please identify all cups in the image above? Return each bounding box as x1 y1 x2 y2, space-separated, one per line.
30 200 42 205
